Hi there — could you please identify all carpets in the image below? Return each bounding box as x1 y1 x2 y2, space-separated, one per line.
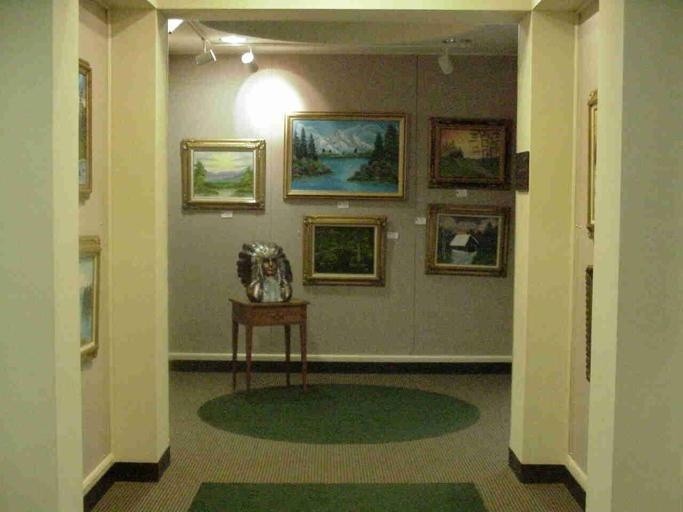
185 482 488 512
197 382 479 446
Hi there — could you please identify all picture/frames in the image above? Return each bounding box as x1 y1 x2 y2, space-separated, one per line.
584 264 591 384
180 138 268 211
78 236 100 362
77 57 95 196
586 88 595 237
283 108 410 201
425 204 506 278
299 214 388 286
426 116 525 192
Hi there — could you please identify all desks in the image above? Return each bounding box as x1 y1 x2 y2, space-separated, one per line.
228 295 309 393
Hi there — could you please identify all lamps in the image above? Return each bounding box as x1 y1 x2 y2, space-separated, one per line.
167 18 473 74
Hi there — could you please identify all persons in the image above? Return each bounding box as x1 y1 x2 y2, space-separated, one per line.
236 242 293 303
